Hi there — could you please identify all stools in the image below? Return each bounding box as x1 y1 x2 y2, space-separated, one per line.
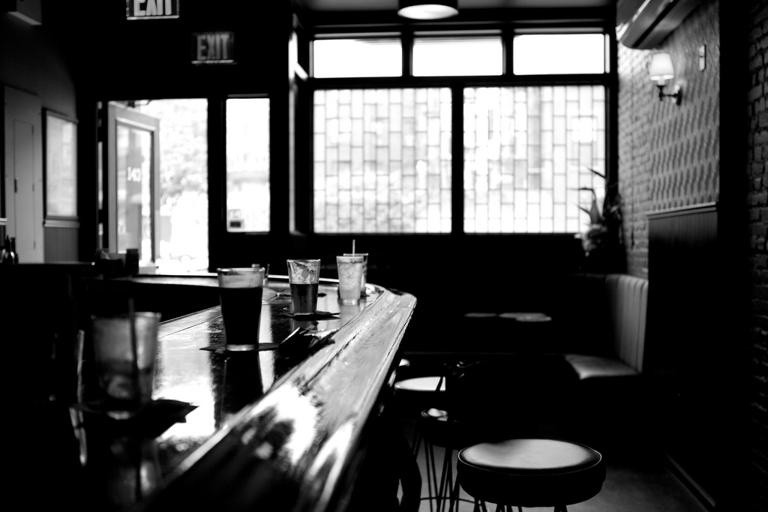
393 376 607 512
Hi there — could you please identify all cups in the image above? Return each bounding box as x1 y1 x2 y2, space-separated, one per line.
219 355 265 422
336 253 369 300
286 258 321 316
105 435 164 509
44 324 88 399
49 404 90 468
87 308 161 419
339 299 361 328
216 265 265 352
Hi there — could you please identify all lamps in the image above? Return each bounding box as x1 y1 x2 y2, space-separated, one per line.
649 53 681 105
397 0 458 20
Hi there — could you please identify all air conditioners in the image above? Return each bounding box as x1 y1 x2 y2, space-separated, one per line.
616 0 696 49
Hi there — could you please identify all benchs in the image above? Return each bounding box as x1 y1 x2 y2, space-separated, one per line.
566 274 651 385
